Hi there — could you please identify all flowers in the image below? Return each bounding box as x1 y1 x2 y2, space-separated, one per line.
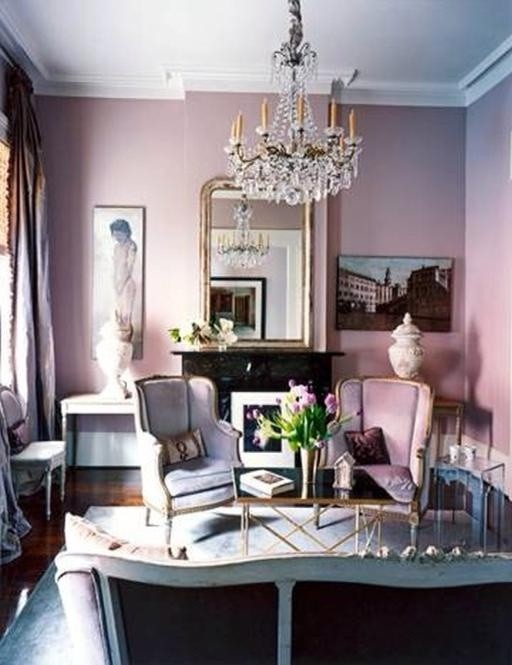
257 380 360 451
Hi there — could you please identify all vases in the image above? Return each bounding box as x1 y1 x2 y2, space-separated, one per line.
299 447 316 499
388 312 427 381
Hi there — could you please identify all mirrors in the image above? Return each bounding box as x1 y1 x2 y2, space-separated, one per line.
200 177 314 351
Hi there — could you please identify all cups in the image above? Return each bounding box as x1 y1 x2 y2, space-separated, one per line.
450 445 460 462
464 444 477 462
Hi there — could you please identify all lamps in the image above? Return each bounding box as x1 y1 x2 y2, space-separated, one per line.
217 193 270 269
223 1 363 207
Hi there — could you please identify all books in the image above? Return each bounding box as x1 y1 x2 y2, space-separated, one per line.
239 469 295 499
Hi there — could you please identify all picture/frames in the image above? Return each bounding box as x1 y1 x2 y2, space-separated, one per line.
335 255 455 333
90 207 144 361
210 277 267 340
231 390 297 470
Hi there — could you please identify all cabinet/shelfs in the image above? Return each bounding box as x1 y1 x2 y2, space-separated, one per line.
171 350 346 422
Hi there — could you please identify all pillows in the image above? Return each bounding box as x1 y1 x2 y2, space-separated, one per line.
64 512 188 562
344 427 391 465
9 415 32 454
153 427 208 466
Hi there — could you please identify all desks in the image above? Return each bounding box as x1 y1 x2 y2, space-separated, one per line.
432 400 464 459
59 394 149 467
434 453 505 553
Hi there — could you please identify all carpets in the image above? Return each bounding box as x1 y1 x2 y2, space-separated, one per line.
0 503 512 665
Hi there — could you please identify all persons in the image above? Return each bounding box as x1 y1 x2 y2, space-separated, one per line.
110 219 139 352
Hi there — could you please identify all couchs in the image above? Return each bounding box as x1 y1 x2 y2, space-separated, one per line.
56 547 512 665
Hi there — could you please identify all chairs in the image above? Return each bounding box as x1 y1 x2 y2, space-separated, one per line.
313 377 434 556
134 376 243 547
0 385 66 520
